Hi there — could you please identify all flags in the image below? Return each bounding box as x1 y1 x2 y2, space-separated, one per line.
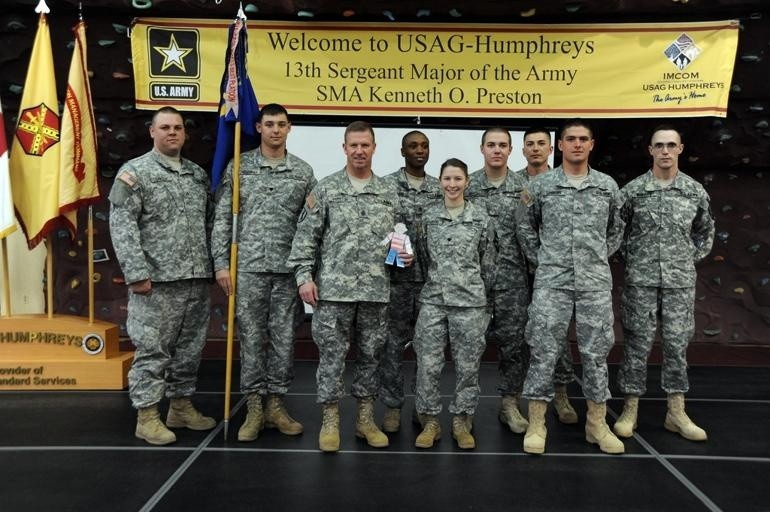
211 8 260 196
9 13 60 251
57 21 105 247
0 100 18 241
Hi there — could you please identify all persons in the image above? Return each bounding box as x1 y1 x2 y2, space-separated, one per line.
412 158 492 449
613 125 715 442
210 104 318 442
285 121 412 452
516 122 625 454
108 106 217 445
513 128 578 425
379 130 443 433
463 126 532 434
